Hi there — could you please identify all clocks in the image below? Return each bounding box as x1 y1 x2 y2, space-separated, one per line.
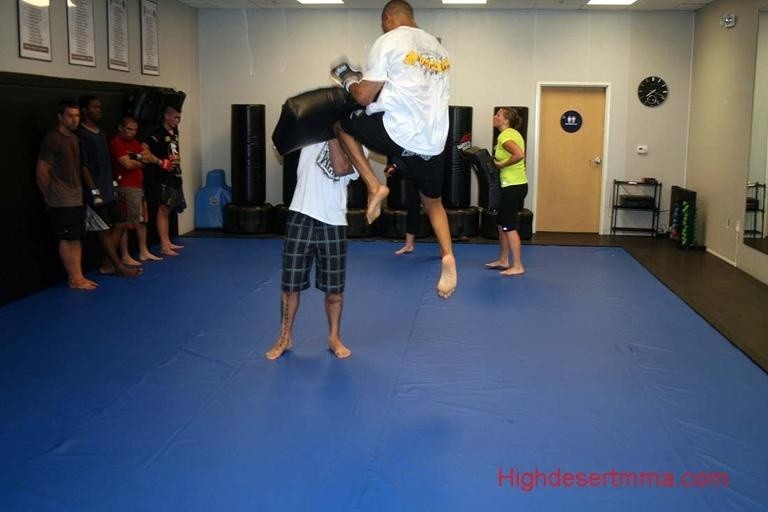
637 76 669 108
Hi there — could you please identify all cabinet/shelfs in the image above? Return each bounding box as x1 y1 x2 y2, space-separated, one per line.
744 181 766 241
611 178 663 238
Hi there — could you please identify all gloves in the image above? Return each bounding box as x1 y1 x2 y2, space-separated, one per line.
329 63 363 92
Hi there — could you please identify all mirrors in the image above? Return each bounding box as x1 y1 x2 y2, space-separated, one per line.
742 11 768 254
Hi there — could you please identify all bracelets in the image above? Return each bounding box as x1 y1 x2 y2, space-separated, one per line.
272 146 276 151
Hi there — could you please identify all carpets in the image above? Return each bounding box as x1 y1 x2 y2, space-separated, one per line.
0 237 766 511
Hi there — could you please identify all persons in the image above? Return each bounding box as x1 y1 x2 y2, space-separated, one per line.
269 126 373 360
485 105 529 277
384 167 420 258
109 117 164 268
76 100 144 280
37 102 98 291
140 105 185 255
332 1 458 298
